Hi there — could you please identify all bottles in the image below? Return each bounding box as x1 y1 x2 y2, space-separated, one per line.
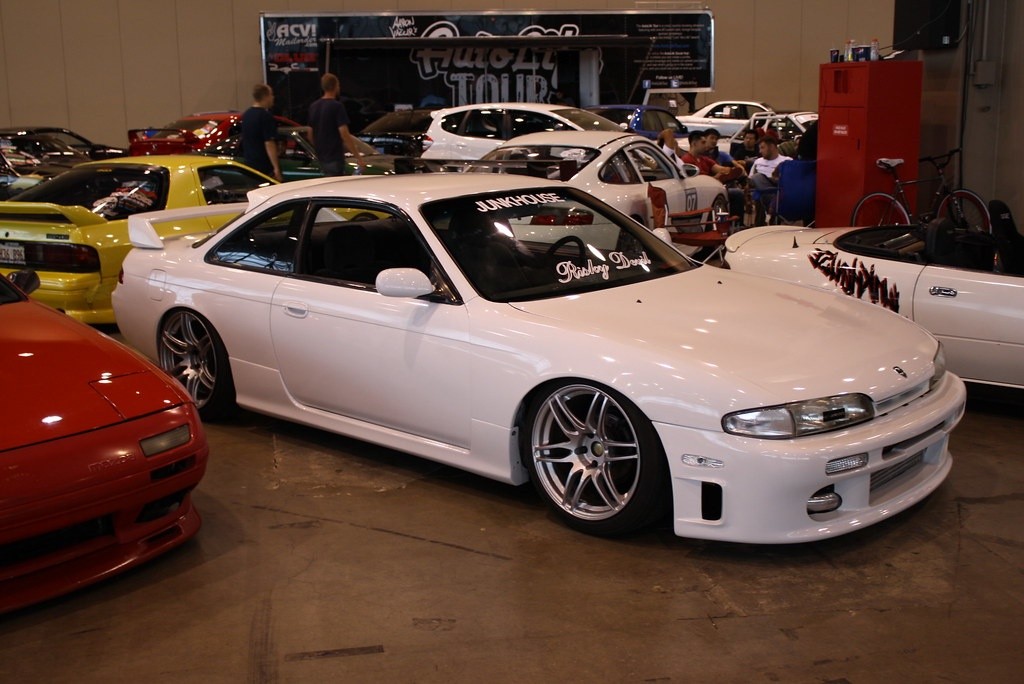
844 39 855 60
871 39 879 60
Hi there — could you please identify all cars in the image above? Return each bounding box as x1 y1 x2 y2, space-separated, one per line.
194 123 396 185
0 269 209 619
356 107 436 174
674 100 777 138
0 126 130 203
0 156 396 324
461 130 730 261
127 112 309 158
583 104 691 140
660 112 820 161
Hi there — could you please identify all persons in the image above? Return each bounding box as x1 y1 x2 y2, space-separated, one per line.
656 128 688 160
231 82 283 182
604 128 657 184
733 129 761 188
307 73 366 177
770 119 818 225
681 130 730 180
744 134 803 223
555 91 575 106
703 128 751 227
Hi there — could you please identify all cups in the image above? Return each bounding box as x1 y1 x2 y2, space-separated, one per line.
830 49 839 62
852 47 870 62
717 212 730 221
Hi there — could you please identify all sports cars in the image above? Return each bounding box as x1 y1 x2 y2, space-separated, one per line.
109 171 967 547
723 199 1024 390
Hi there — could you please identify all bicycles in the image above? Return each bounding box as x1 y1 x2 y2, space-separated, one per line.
850 148 992 267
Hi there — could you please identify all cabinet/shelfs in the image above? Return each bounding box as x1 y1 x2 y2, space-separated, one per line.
816 60 923 228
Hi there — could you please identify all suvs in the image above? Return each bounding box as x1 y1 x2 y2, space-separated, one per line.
419 102 658 174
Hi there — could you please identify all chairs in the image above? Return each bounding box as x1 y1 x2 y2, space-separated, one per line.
988 199 1024 274
927 217 956 263
449 211 530 293
313 225 395 284
647 160 817 267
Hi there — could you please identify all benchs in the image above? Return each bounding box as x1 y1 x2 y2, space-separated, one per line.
254 218 431 269
